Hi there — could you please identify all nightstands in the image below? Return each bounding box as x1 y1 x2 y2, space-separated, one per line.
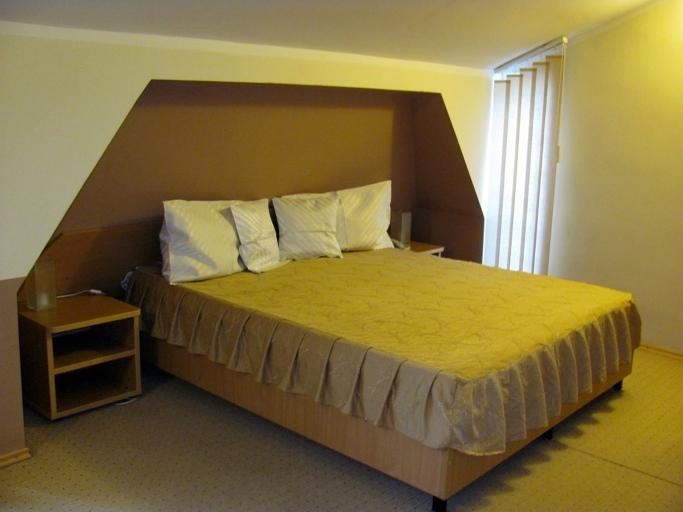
392 240 445 257
17 292 142 421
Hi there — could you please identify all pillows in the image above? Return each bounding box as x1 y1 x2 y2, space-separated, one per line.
272 189 344 260
159 199 247 285
336 179 394 253
230 200 292 275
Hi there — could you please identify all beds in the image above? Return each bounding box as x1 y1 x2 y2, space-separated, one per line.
126 249 642 510
